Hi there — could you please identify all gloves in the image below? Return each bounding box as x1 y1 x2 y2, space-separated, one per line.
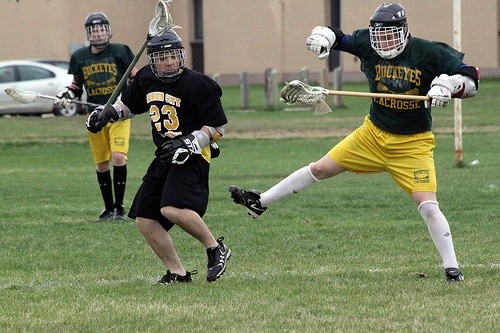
158 132 201 169
85 104 122 134
424 74 455 111
53 87 77 117
306 25 336 59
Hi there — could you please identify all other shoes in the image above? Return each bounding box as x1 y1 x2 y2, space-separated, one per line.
113 206 126 220
96 209 114 222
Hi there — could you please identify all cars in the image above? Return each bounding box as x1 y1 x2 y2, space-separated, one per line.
0 59 88 117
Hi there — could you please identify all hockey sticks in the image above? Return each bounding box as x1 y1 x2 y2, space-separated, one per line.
280 79 429 107
97 0 174 120
5 87 103 108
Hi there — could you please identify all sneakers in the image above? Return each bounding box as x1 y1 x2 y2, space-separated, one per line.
227 186 269 220
154 269 198 288
206 236 232 283
445 268 465 286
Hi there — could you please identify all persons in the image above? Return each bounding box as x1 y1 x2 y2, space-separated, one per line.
54 12 138 220
229 3 478 283
86 29 232 284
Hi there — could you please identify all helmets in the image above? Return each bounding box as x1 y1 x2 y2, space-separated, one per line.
145 28 186 84
83 13 113 50
369 2 410 59
